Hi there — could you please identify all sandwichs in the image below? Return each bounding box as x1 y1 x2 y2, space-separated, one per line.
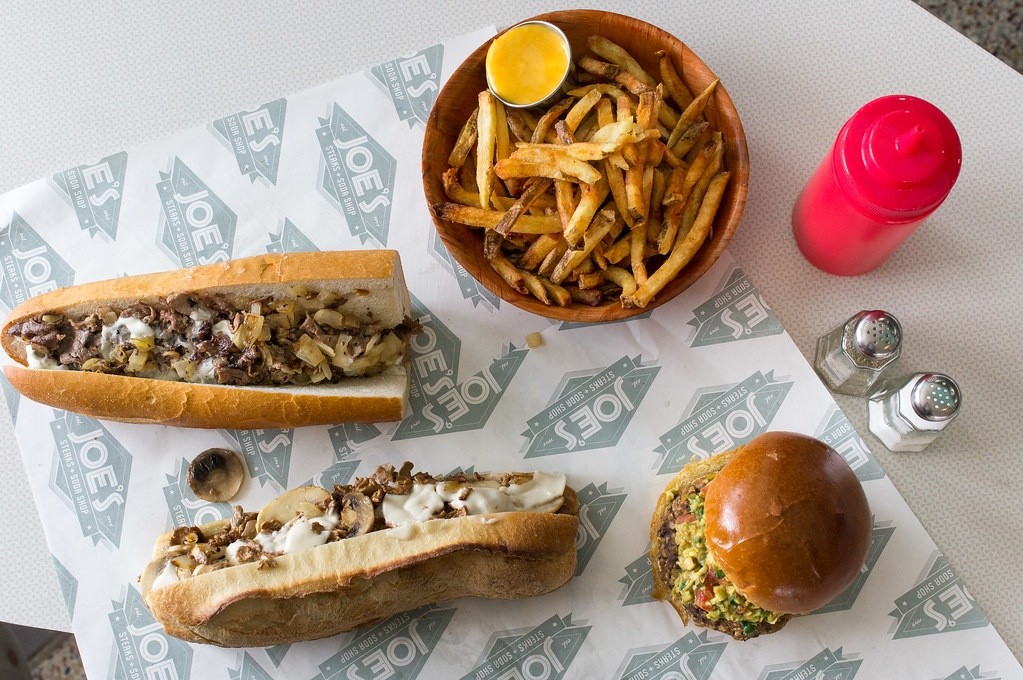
0 248 422 424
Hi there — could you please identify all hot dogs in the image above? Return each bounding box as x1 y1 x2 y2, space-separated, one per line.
136 462 581 648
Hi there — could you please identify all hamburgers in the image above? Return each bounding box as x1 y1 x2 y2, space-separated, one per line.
650 431 873 640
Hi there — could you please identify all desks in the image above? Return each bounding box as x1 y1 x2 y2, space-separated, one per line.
0 0 1023 668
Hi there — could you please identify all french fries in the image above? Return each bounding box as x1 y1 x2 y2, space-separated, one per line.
441 36 732 309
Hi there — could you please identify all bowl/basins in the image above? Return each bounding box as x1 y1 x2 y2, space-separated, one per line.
421 8 749 324
485 20 572 108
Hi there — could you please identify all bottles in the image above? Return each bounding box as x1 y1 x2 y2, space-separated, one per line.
864 371 961 453
814 309 903 397
791 95 962 276
